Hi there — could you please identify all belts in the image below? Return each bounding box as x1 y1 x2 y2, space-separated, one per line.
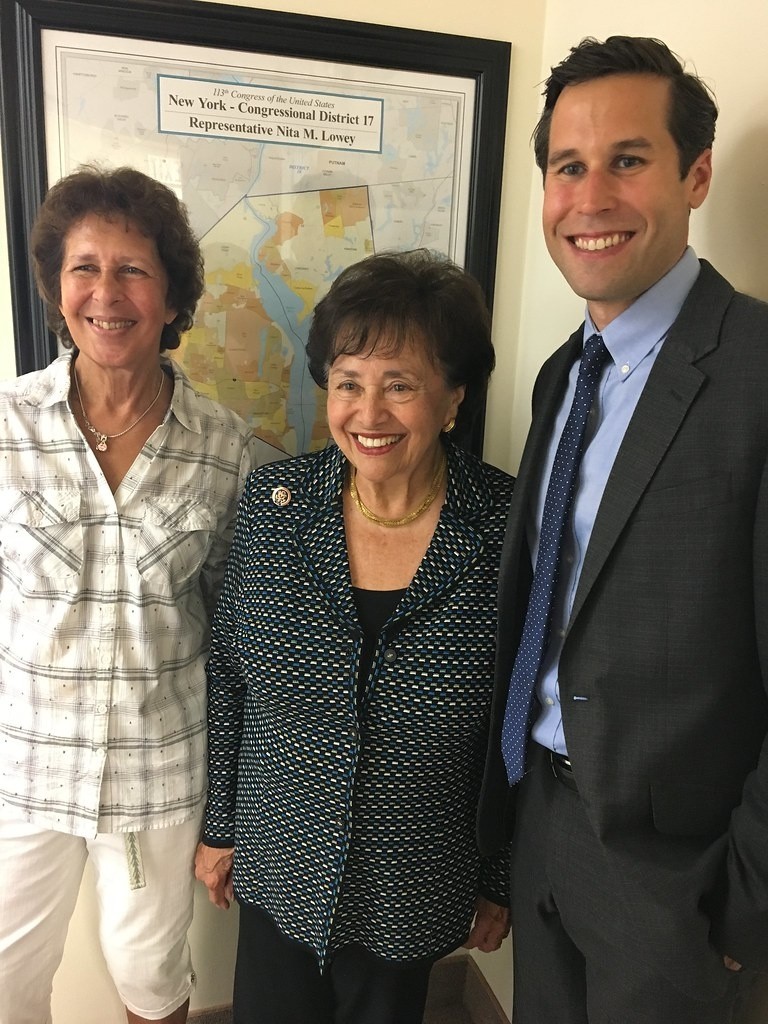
546 752 577 787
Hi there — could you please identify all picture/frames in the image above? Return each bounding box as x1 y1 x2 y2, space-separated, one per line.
1 0 512 472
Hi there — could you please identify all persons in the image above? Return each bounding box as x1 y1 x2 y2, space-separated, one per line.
196 247 516 1024
477 36 768 1024
0 164 252 1024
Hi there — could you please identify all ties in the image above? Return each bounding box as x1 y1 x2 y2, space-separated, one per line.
500 334 614 785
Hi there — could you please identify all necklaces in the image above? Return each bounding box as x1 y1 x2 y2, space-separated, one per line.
348 455 448 529
72 367 164 452
